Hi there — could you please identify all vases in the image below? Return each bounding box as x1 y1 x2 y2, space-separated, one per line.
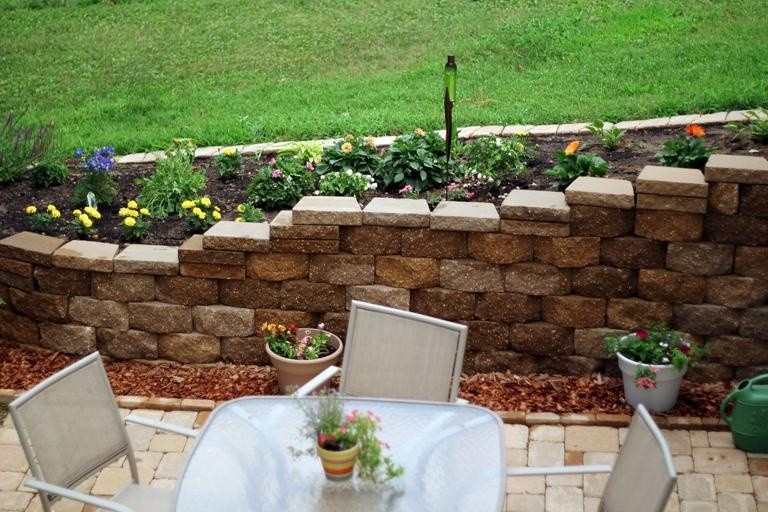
264 329 342 394
317 444 361 481
613 334 689 413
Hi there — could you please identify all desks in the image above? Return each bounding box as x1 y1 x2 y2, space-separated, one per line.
173 396 508 512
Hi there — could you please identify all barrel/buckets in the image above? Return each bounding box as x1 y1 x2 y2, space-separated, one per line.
720 373 767 452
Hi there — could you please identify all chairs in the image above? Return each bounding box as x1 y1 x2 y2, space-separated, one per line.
9 349 199 512
507 405 677 512
292 299 472 403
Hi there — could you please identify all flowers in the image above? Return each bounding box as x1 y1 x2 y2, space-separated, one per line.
295 391 399 483
258 321 330 357
599 328 701 388
